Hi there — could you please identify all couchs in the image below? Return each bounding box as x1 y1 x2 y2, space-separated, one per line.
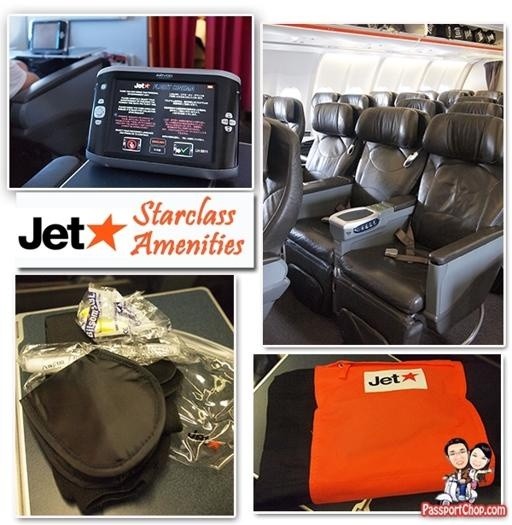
264 97 306 143
284 107 431 315
311 90 504 121
299 103 362 187
328 113 503 344
262 115 303 320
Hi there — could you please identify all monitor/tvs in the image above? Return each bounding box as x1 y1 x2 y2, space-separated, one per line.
102 78 215 162
31 20 67 53
